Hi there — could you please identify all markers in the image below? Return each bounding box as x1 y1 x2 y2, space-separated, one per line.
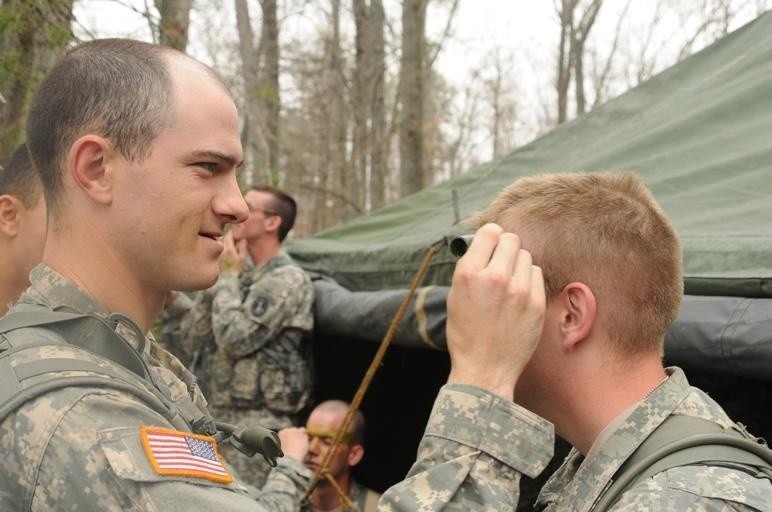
451 235 479 255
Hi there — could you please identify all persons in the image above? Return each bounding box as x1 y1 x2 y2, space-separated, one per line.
477 168 771 512
299 401 386 509
1 141 50 316
260 423 314 503
182 186 315 479
0 38 546 512
158 290 210 404
